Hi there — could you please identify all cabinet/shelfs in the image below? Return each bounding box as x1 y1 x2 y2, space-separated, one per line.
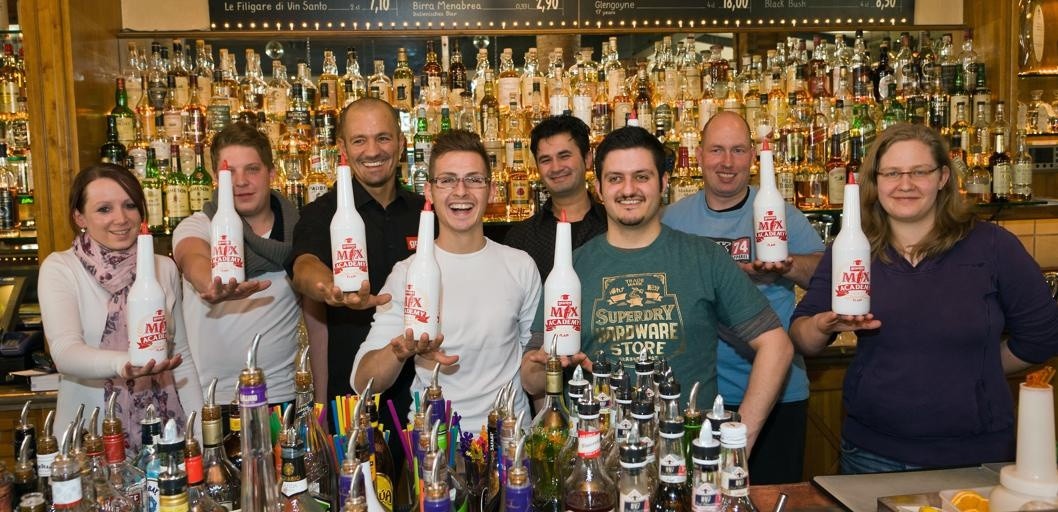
966 0 1054 270
0 1 125 271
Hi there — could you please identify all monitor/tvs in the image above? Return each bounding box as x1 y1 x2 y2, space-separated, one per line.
0 275 28 332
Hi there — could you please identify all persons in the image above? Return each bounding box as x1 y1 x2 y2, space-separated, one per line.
657 107 826 484
171 127 330 443
348 129 545 454
290 97 437 436
36 162 209 461
518 129 794 473
787 122 1055 475
496 114 610 279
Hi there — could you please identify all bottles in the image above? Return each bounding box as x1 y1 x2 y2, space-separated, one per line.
1027 88 1058 135
100 29 1036 228
208 160 246 285
5 332 760 512
401 196 443 342
121 216 170 369
543 205 581 357
830 169 872 315
329 150 372 292
0 25 39 238
752 140 789 264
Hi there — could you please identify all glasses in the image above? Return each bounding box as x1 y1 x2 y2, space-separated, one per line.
876 165 938 180
429 175 491 189
